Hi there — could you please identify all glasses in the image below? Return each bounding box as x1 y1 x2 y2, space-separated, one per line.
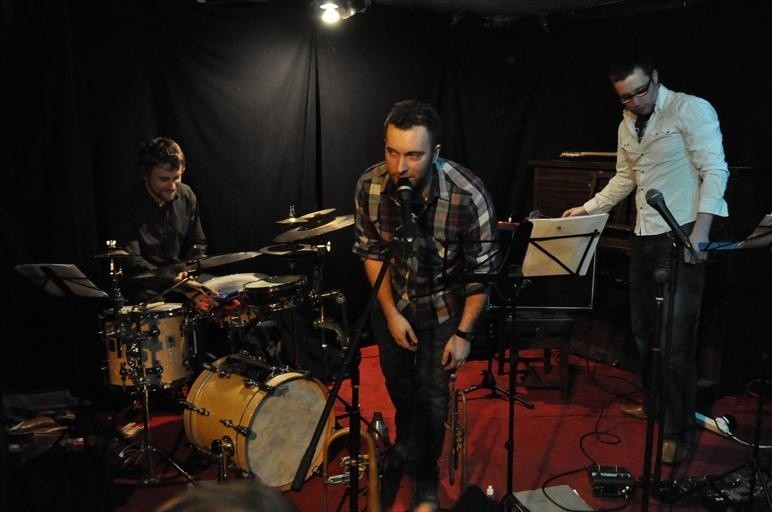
617 71 654 105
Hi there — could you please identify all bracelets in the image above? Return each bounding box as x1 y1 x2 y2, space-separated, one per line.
452 326 478 345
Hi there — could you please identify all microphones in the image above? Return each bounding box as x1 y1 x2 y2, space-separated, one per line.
394 178 417 239
645 188 696 255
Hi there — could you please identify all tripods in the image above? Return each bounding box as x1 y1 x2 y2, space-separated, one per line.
458 277 535 409
496 282 530 512
106 375 199 488
668 249 771 511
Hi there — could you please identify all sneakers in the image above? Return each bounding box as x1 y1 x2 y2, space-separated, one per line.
411 478 440 512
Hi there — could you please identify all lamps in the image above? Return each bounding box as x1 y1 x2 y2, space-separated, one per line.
311 1 370 24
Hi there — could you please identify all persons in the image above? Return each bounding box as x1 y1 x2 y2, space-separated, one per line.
116 137 221 414
353 97 501 511
561 57 730 467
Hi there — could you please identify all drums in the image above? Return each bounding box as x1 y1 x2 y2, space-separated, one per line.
180 355 336 489
201 270 255 296
246 275 310 314
98 299 191 386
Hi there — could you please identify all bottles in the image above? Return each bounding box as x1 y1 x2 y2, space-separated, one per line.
484 483 496 499
367 409 389 444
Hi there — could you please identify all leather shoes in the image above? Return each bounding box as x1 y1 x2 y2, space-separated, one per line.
662 430 693 466
618 402 649 419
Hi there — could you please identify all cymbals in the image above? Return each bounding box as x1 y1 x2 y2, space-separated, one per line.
302 208 334 219
131 250 259 276
275 216 308 227
185 249 261 273
271 215 356 242
96 248 128 256
259 243 316 259
